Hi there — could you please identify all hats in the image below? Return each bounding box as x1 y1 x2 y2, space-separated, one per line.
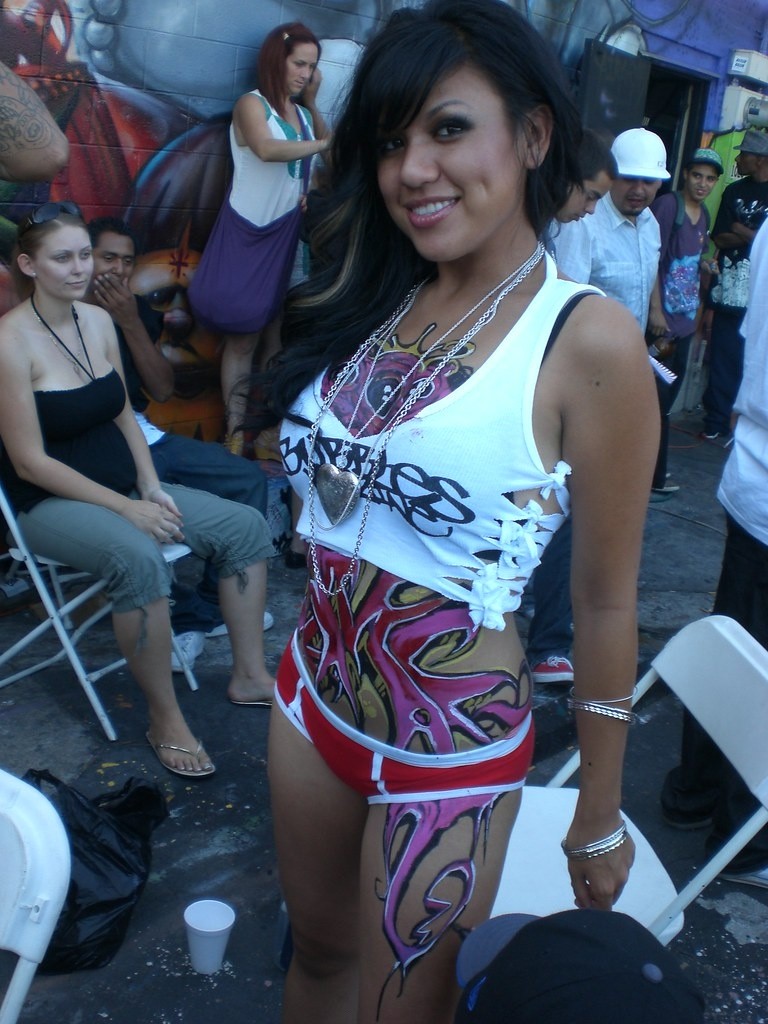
733 129 768 155
457 908 708 1023
611 127 671 180
685 147 725 175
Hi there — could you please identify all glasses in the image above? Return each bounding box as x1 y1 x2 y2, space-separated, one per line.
21 200 82 238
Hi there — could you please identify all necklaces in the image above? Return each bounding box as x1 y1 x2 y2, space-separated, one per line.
32 305 83 372
307 241 548 594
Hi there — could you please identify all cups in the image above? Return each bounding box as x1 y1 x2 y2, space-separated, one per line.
184 900 235 974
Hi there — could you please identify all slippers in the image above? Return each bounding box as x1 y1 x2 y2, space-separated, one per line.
145 732 216 777
232 699 273 707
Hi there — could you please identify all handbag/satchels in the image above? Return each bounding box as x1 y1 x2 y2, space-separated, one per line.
24 769 172 976
186 197 306 331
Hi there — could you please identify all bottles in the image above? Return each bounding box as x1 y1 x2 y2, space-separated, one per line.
649 333 681 361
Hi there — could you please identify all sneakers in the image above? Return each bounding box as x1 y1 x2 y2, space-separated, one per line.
531 657 573 682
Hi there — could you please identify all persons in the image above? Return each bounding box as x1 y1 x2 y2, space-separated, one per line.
667 210 768 876
540 129 768 493
220 22 333 454
0 60 276 778
267 0 662 1024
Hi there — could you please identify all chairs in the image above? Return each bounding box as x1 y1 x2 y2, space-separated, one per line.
488 615 768 947
0 767 72 1024
0 488 198 739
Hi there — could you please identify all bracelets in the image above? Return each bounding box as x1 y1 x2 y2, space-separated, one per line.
561 821 628 861
566 686 637 724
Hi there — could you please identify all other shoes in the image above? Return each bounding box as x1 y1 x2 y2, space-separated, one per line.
650 478 680 491
722 864 768 887
663 810 712 828
702 427 718 439
